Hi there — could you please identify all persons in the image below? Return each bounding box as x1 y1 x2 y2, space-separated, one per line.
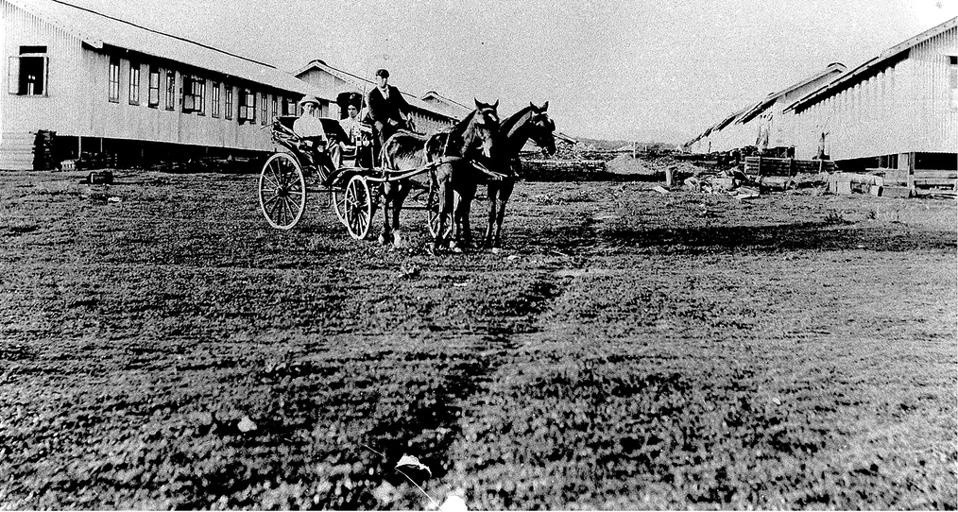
364 69 412 170
292 93 331 156
337 103 373 170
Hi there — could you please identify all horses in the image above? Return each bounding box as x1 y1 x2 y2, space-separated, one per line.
376 97 502 255
428 100 557 252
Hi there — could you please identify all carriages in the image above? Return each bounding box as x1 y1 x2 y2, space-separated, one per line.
257 96 555 257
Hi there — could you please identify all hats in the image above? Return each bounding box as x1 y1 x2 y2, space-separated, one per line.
297 95 321 108
